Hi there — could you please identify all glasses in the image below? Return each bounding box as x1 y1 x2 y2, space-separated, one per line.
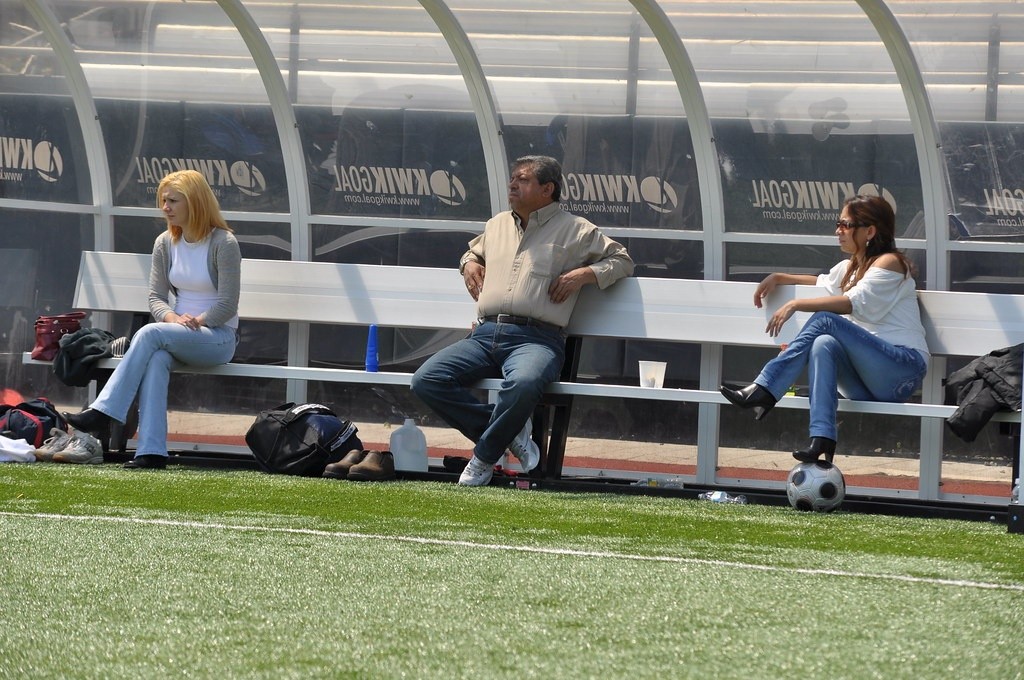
836 221 872 230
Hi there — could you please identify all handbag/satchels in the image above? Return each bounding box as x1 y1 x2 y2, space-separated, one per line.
32 310 86 361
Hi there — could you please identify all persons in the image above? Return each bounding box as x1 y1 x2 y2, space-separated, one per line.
410 155 635 486
718 194 932 463
62 170 242 469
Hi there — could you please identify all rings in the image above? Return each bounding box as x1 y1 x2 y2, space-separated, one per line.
468 285 471 289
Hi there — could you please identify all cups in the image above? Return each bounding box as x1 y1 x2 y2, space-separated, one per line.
638 360 667 388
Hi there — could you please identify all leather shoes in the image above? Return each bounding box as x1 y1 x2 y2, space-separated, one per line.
124 453 166 470
63 407 111 434
346 449 396 479
323 447 369 477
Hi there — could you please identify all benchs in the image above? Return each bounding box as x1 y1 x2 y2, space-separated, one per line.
22 250 1024 505
79 1 1023 128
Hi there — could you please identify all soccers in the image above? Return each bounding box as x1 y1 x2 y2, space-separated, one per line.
786 460 846 514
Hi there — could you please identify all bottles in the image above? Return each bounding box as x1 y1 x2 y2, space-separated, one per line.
649 478 684 490
1010 478 1020 504
777 344 796 397
365 324 379 372
390 419 428 473
698 491 747 505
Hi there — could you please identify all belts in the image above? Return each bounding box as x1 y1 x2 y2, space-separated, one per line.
481 313 566 336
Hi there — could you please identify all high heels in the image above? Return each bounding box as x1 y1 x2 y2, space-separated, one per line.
718 382 778 421
793 436 837 466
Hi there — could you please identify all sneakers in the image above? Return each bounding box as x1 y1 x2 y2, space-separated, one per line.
34 435 78 461
507 417 539 472
457 456 495 486
52 434 104 463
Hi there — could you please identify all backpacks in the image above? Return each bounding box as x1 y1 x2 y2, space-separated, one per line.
0 398 68 448
245 401 363 476
